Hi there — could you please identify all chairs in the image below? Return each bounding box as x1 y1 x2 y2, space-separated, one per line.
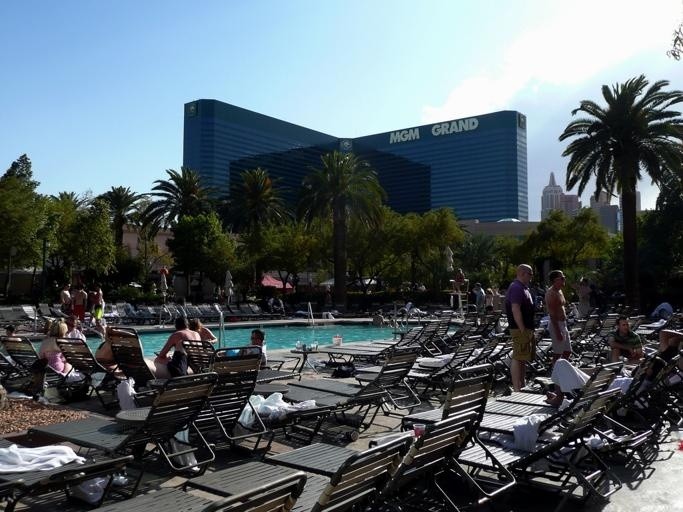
0 298 683 512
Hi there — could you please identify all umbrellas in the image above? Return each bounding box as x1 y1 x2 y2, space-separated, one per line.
158 266 167 297
223 271 234 297
443 246 455 273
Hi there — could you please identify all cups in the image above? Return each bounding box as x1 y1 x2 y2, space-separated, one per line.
412 423 425 437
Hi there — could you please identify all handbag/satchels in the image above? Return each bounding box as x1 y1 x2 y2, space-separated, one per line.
331 364 357 379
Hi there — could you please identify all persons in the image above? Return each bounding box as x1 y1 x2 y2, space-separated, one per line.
471 283 487 316
504 264 535 392
60 280 71 315
577 277 592 316
491 285 504 335
403 301 425 314
4 324 17 338
655 327 682 361
544 270 571 364
451 268 464 292
70 281 87 323
37 319 75 375
187 318 218 347
542 355 668 407
217 328 267 365
266 294 285 314
484 288 494 315
142 316 203 380
89 281 106 335
62 314 88 345
370 309 386 328
606 314 644 364
94 325 120 379
